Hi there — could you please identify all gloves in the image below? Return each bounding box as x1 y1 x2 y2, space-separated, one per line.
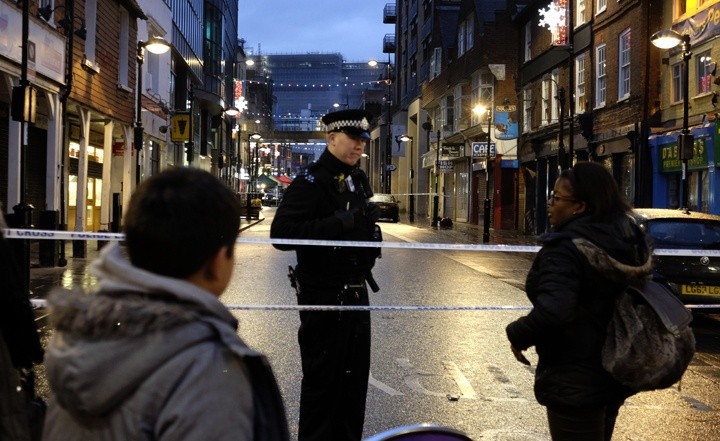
352 203 379 227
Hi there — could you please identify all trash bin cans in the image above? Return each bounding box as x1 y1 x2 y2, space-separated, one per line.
39 211 58 266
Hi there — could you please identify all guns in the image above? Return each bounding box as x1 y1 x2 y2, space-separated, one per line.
366 270 380 294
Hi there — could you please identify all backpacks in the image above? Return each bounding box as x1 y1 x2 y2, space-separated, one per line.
573 275 698 390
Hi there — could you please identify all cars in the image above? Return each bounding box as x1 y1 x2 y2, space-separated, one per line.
262 186 284 204
370 193 400 223
630 208 720 327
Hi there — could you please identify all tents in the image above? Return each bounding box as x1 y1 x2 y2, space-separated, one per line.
257 174 292 208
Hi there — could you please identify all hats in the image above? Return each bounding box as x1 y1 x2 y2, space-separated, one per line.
322 109 372 141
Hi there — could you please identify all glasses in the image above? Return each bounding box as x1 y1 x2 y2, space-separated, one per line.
549 189 582 207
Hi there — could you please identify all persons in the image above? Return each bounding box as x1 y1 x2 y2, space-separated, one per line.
41 165 293 441
504 162 654 441
270 110 379 441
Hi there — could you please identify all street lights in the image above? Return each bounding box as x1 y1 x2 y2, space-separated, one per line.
474 105 493 243
648 28 690 210
367 54 391 193
228 59 255 176
245 133 263 223
217 106 240 177
398 135 414 223
134 36 172 185
